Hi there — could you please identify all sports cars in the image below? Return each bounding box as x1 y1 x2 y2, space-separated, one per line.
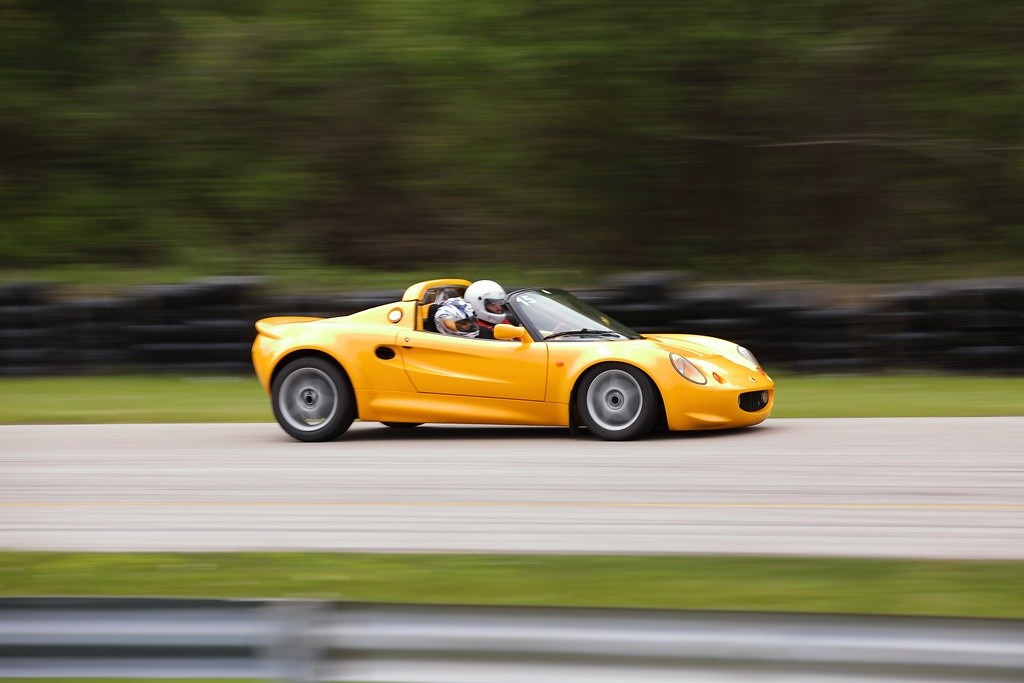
251 278 775 442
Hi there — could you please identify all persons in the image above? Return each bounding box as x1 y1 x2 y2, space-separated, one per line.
434 280 563 342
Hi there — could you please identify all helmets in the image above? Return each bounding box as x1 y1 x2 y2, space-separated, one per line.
434 297 480 339
463 279 508 323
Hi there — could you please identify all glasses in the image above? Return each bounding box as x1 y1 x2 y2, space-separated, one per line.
455 317 476 325
